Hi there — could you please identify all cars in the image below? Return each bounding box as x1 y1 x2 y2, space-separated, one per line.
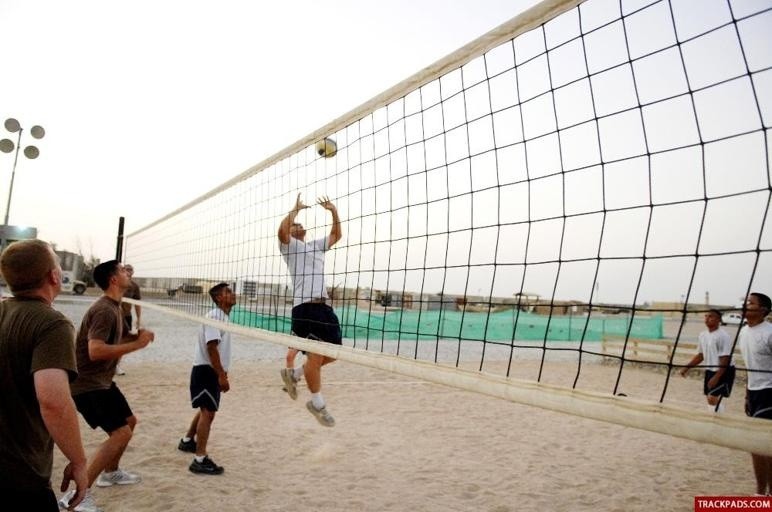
721 313 748 326
60 271 86 294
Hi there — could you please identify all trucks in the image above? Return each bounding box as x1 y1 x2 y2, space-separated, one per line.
168 283 203 296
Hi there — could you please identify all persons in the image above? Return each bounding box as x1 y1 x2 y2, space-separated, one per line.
55 260 154 511
277 192 342 427
114 264 141 376
679 309 736 413
1 238 89 512
175 282 237 474
738 293 772 494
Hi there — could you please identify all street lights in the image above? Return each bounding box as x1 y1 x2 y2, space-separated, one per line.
0 118 45 225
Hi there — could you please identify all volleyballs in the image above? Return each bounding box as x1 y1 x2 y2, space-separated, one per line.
317 138 336 160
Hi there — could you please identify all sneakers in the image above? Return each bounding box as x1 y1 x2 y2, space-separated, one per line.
306 401 336 427
281 368 301 400
97 466 141 488
179 437 196 452
114 365 124 376
189 455 223 475
58 487 102 512
